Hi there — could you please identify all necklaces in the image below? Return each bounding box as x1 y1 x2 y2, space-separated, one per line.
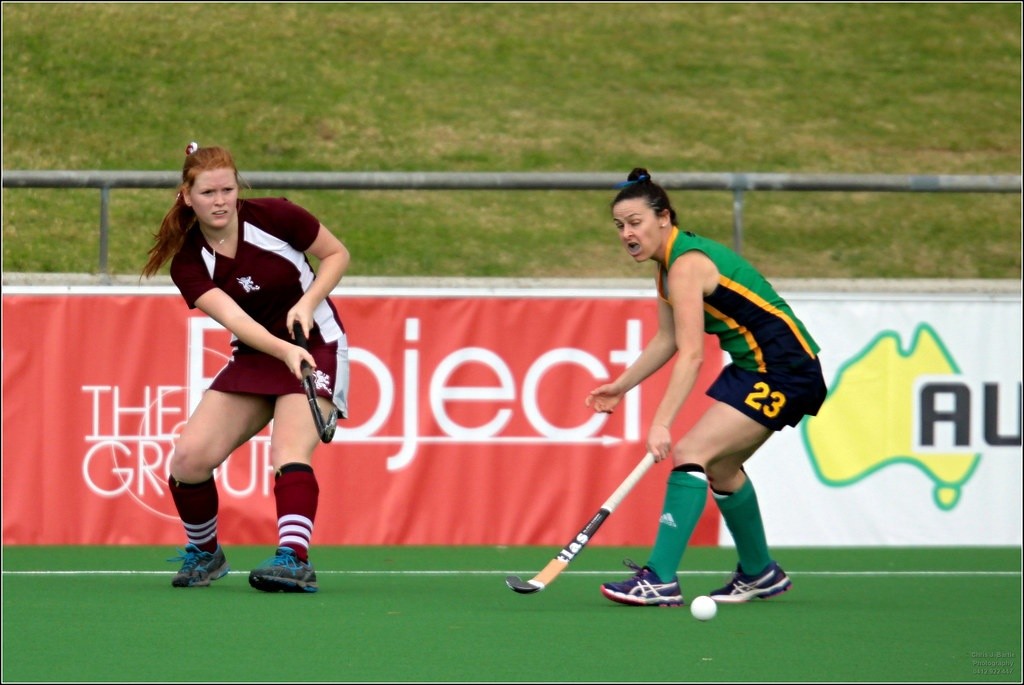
200 224 237 260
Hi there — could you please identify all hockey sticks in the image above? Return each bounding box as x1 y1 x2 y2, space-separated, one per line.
505 453 654 594
293 320 339 444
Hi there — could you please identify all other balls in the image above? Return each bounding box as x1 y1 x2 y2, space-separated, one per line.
689 594 717 623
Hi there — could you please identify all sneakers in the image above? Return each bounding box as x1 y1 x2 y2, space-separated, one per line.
600 558 684 607
710 560 792 602
249 546 320 593
166 543 230 587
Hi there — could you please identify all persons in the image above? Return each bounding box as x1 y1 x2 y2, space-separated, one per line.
139 143 350 592
584 169 826 606
